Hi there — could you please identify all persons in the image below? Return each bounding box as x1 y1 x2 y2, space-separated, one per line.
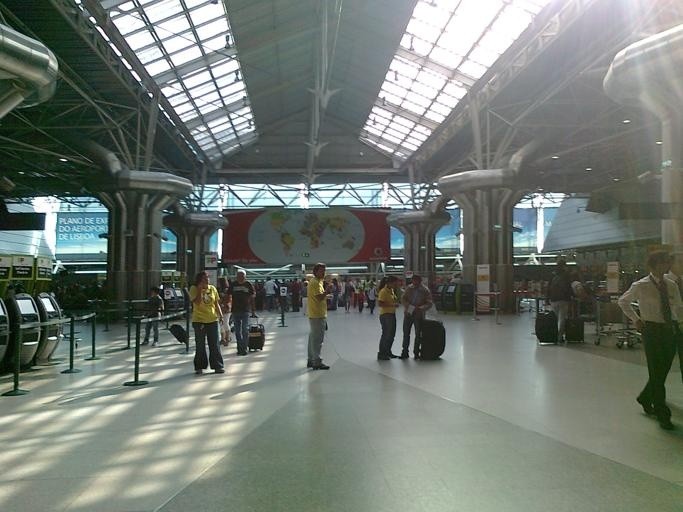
399 275 432 360
546 260 575 344
140 287 164 346
251 277 379 314
378 276 400 360
224 269 256 355
664 252 683 385
216 278 232 347
189 272 225 374
618 252 683 429
306 263 334 370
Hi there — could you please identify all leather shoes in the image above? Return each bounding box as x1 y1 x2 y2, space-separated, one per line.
195 368 226 374
308 359 329 369
637 395 674 430
378 352 409 360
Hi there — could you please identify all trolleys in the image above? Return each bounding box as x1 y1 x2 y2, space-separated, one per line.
588 292 646 350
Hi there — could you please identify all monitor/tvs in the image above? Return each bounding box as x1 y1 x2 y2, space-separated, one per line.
41 297 57 312
16 298 37 315
0 300 6 315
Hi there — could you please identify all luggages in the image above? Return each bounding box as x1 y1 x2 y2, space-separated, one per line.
159 318 187 343
247 315 265 351
419 311 445 360
535 271 584 343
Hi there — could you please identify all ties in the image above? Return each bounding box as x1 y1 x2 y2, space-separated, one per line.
650 275 670 322
